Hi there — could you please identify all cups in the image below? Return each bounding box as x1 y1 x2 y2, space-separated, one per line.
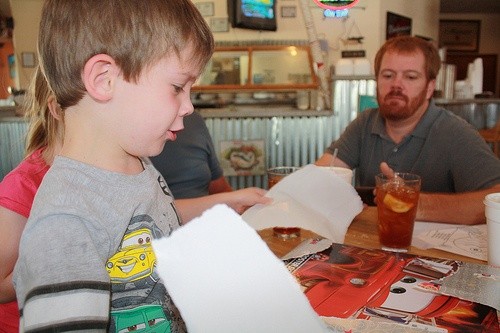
482 193 500 269
375 172 421 252
267 167 302 238
324 167 353 185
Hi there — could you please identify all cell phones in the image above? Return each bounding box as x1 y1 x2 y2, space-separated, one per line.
354 187 376 206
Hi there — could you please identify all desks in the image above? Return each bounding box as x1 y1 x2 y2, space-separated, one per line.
259 207 500 333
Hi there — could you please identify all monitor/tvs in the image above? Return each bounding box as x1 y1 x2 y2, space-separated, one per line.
228 0 277 31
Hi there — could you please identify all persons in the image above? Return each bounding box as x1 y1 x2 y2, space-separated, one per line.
1 66 65 302
150 110 284 200
307 36 500 226
13 0 215 333
0 64 275 333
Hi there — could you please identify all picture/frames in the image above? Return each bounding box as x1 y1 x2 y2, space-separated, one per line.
386 10 412 40
438 19 482 52
